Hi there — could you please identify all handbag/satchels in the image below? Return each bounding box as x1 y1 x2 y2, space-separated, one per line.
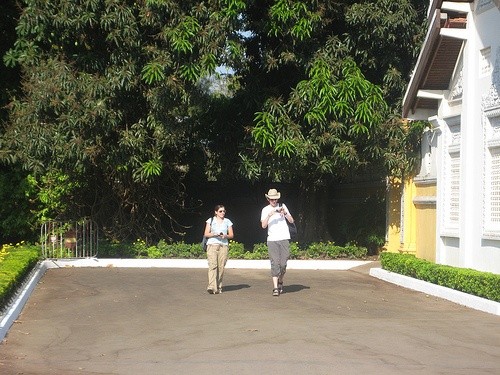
200 217 212 253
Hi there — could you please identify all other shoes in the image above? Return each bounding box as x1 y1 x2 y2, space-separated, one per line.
207 287 215 294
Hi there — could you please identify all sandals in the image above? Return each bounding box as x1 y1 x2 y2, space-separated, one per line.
278 278 284 294
272 288 280 296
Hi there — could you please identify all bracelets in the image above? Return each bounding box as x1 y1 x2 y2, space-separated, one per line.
284 212 288 216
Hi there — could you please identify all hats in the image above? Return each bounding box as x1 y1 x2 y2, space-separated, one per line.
265 189 281 199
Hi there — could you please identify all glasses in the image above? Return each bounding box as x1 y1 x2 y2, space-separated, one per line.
219 210 226 213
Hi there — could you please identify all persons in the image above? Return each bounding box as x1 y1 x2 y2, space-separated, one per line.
204 205 234 294
260 189 294 296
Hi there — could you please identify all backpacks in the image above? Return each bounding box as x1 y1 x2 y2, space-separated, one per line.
279 202 297 243
